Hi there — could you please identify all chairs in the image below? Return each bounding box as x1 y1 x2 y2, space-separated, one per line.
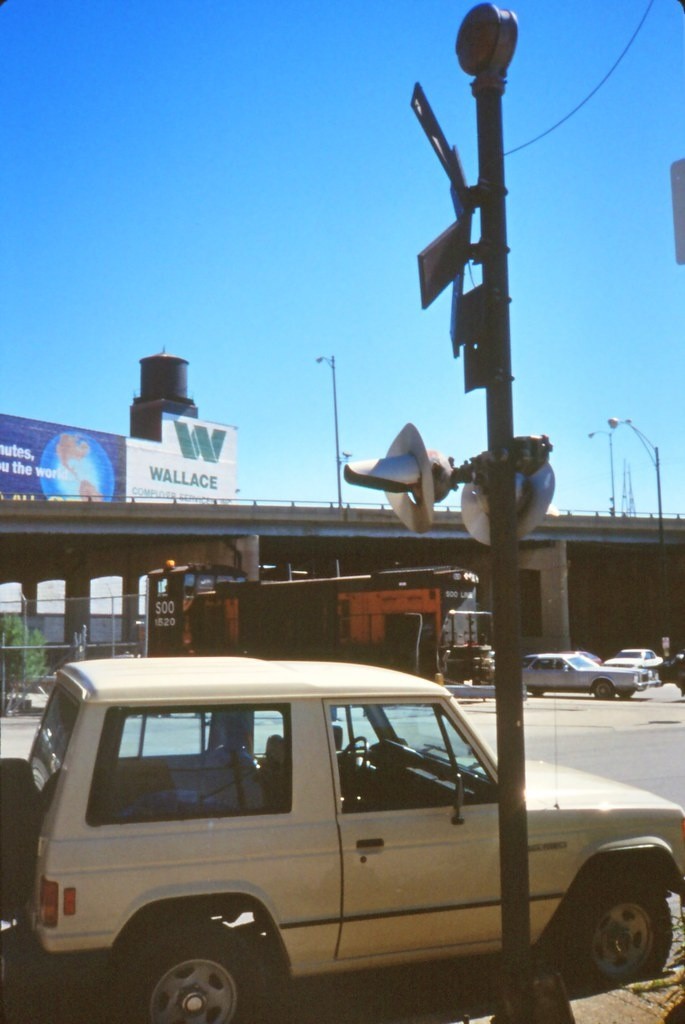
199 715 260 812
260 735 292 812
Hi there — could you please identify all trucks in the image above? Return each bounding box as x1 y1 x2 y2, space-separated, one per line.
132 554 500 704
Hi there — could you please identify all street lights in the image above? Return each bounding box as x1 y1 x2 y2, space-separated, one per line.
315 356 354 508
609 418 671 660
587 429 615 515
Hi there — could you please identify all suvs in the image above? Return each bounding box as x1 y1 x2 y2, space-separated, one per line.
1 654 684 1024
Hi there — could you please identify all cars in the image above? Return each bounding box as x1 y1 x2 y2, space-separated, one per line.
518 653 663 699
604 648 663 677
